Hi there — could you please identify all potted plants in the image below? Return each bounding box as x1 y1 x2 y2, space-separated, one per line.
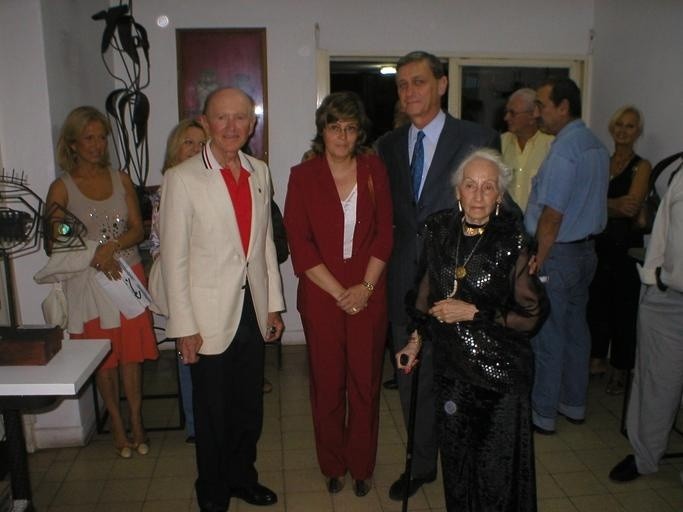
91 1 161 239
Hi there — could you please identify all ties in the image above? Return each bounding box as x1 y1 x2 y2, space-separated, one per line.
410 131 425 202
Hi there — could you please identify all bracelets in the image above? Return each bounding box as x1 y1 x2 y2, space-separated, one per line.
113 240 121 251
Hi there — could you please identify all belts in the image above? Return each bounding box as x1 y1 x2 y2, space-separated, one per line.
569 234 597 244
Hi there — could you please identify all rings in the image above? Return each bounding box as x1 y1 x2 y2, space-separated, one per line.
176 350 183 359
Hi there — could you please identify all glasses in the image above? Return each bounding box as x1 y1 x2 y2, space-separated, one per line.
503 108 532 117
330 125 359 134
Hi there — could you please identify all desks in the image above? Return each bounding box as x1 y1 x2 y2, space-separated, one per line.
0 340 112 512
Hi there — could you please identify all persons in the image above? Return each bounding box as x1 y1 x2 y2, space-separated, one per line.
146 117 218 445
283 49 550 512
41 105 167 460
491 77 681 483
154 83 287 512
